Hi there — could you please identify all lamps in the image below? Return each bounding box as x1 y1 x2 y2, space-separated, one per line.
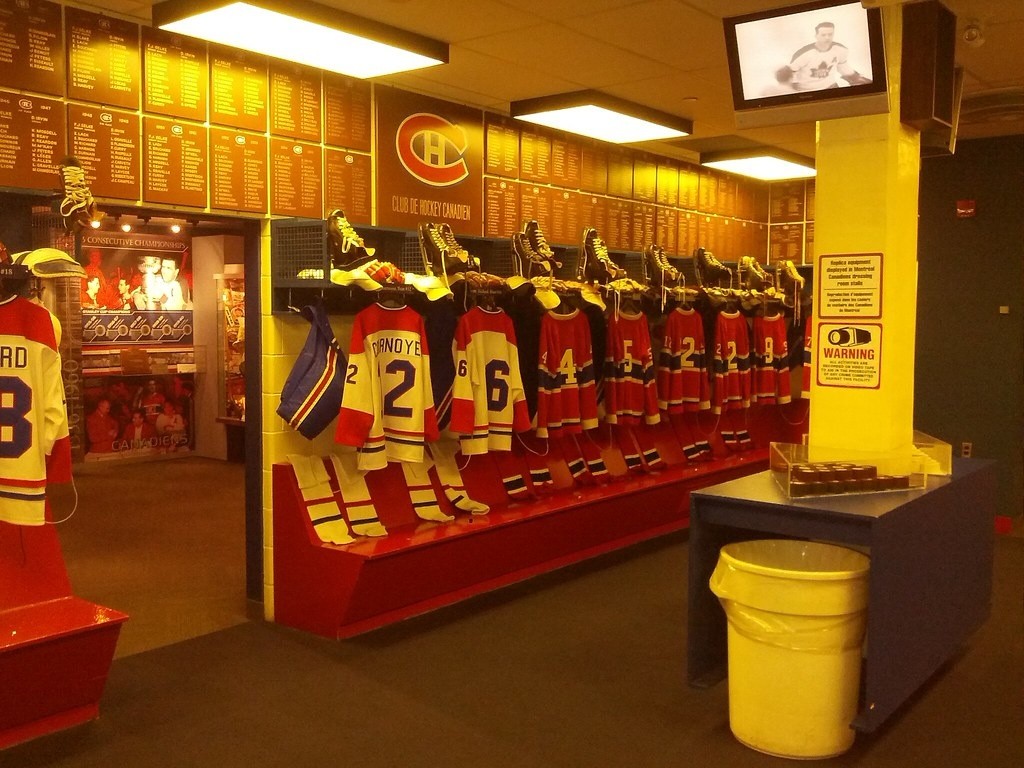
152 0 449 80
700 145 816 180
510 88 693 144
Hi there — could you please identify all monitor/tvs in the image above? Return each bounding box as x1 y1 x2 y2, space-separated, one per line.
919 68 966 158
723 0 890 130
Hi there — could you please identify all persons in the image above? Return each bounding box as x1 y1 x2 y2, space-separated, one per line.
87 381 187 452
761 65 800 95
790 23 871 91
82 249 194 311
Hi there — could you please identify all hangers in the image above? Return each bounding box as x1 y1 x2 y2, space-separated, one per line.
620 298 640 314
376 291 406 306
478 294 499 312
724 301 737 313
678 301 692 310
554 295 576 313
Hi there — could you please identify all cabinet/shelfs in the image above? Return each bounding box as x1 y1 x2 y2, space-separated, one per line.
244 218 814 643
0 186 129 749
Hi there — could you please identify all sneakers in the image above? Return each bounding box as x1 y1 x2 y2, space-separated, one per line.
737 256 773 293
327 208 375 272
751 257 774 287
511 232 551 283
693 247 721 290
644 244 676 313
777 260 801 326
601 239 627 322
524 220 562 291
786 261 806 293
418 222 468 312
708 252 732 296
577 228 617 298
435 223 480 307
660 247 685 305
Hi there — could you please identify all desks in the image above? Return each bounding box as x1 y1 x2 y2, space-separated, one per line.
685 459 998 735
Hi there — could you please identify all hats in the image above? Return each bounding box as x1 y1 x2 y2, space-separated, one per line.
350 269 383 292
413 276 454 301
507 276 531 290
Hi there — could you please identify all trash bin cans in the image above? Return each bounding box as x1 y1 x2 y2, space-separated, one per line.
708 539 872 761
